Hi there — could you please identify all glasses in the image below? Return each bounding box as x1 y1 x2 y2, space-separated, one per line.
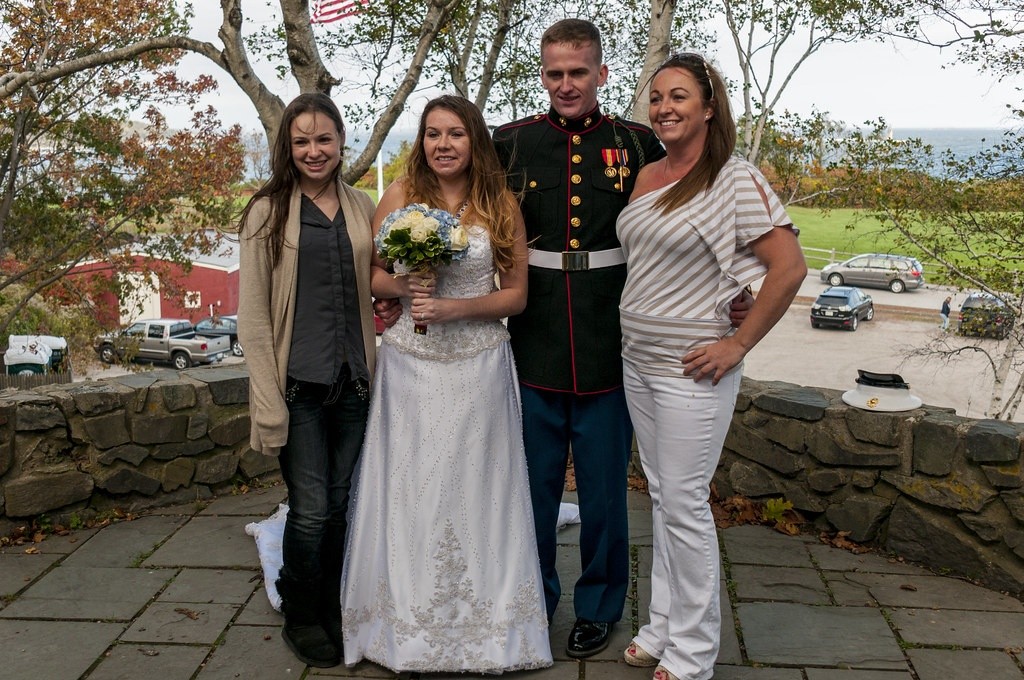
662 53 715 98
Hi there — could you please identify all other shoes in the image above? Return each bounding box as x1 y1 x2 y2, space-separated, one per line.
653 660 680 680
623 640 659 666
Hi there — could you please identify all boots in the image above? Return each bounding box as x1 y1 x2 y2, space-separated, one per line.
275 566 345 669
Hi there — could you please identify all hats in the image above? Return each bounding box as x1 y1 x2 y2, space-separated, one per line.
842 369 923 412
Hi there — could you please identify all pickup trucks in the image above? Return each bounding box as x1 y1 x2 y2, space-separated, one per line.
93 319 232 371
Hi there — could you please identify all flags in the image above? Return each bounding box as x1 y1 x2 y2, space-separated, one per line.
308 0 371 23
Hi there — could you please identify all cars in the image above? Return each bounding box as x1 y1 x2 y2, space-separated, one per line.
194 315 246 358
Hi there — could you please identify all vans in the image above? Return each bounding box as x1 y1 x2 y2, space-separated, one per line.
822 252 924 293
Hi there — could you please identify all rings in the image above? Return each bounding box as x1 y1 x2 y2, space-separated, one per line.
420 313 424 321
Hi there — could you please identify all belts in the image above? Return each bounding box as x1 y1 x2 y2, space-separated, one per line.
528 248 626 271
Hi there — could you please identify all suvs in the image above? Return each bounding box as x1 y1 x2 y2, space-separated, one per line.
811 285 875 332
959 291 1016 341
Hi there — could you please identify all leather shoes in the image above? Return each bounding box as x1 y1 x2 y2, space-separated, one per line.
566 618 615 657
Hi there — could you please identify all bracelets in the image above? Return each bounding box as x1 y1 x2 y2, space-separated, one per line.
744 285 752 294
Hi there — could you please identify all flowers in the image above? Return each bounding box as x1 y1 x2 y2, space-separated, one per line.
373 202 471 335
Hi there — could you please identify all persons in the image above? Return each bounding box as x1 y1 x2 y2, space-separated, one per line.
236 91 376 669
340 95 555 676
372 18 753 659
615 53 807 679
941 297 952 333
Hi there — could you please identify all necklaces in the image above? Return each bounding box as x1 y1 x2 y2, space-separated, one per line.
662 157 668 186
448 199 469 227
